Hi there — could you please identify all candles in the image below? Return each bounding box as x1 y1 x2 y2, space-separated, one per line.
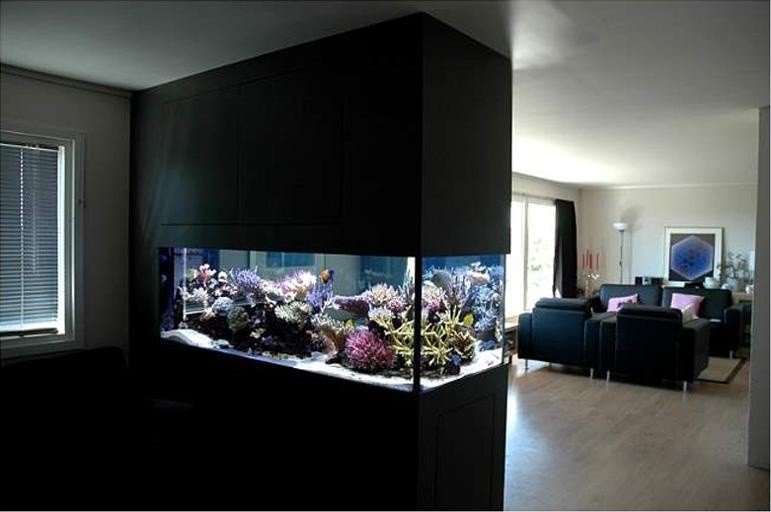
580 249 598 272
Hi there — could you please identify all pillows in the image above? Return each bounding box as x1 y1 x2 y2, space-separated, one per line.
606 293 638 314
670 292 705 324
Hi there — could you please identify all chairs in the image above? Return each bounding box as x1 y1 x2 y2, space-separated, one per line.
516 298 710 393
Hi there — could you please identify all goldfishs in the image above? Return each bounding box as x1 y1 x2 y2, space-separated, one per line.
318 268 335 284
186 268 199 282
463 314 474 326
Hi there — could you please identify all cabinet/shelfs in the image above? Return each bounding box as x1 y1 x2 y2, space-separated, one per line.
503 322 518 365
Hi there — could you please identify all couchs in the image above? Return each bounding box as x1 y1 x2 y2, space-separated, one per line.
588 283 751 361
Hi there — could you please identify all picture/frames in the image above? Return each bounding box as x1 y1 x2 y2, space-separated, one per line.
662 226 723 289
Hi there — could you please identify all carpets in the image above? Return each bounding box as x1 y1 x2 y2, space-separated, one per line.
696 355 747 384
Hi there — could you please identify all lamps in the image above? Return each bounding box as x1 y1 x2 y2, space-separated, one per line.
613 222 628 284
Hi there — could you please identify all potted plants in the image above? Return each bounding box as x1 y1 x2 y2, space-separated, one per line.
717 251 750 291
